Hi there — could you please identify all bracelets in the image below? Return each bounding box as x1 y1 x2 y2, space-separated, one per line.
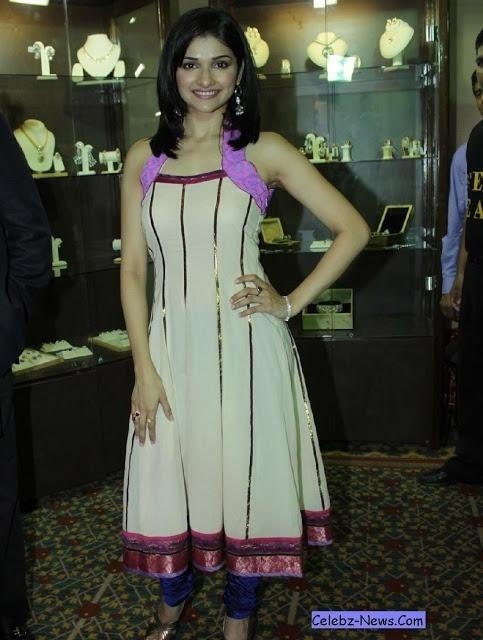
283 295 290 322
315 296 344 313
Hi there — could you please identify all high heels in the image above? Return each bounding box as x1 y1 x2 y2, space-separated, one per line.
144 610 182 640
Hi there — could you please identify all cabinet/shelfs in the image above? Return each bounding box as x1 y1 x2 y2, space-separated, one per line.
0 0 168 510
208 0 449 449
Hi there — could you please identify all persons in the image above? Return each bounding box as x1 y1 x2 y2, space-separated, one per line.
435 70 482 320
114 6 371 640
423 28 481 491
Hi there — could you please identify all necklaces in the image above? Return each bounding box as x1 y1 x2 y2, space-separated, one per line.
312 37 339 58
20 127 48 163
387 23 401 44
248 39 258 55
83 42 113 64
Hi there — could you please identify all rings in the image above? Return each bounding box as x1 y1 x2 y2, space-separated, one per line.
134 411 140 417
145 417 155 423
256 286 262 296
132 414 136 420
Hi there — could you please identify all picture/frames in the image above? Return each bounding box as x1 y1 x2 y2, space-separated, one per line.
377 204 412 233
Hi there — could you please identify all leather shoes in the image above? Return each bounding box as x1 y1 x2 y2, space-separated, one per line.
420 469 458 486
6 624 35 640
